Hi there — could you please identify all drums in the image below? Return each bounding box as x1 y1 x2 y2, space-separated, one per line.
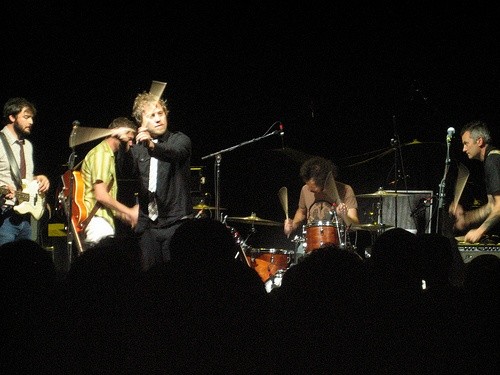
303 220 340 251
246 248 289 284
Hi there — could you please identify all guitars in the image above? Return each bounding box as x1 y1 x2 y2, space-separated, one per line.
57 171 88 254
0 179 46 221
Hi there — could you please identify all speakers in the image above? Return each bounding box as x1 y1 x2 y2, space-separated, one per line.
189 195 207 215
116 179 139 208
190 168 202 193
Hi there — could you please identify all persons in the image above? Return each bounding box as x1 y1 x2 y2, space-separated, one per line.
448 120 500 243
284 156 360 235
0 218 500 375
0 98 50 244
80 117 139 247
128 92 193 243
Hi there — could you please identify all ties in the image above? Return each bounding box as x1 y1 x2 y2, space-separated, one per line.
15 139 27 179
147 139 159 223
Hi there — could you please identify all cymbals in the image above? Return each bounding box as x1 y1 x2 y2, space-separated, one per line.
351 224 394 231
192 203 226 210
227 215 283 226
356 191 412 197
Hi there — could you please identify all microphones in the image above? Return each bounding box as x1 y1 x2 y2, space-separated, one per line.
71 120 81 134
445 127 455 145
